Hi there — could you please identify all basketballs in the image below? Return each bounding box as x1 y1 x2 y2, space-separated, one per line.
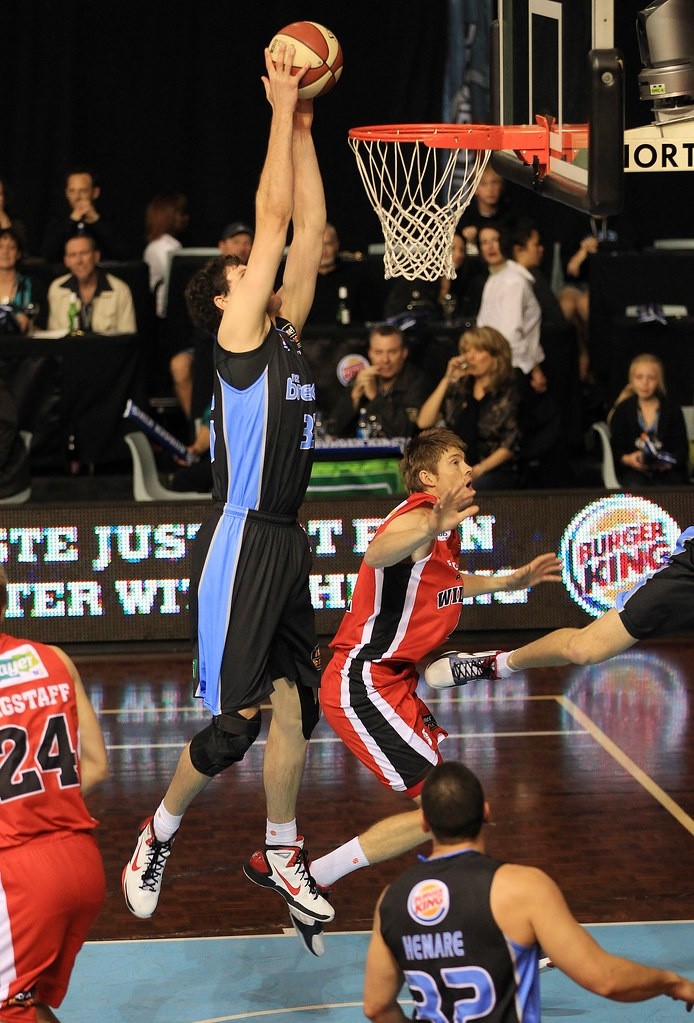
268 21 344 100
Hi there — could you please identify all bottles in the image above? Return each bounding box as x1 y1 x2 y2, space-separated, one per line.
336 287 348 322
357 408 372 441
68 293 82 333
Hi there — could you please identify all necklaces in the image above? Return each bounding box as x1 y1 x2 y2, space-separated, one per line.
0 282 15 304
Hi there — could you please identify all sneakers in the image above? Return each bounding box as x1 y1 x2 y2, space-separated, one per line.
423 650 506 690
119 815 179 919
287 883 334 957
240 836 336 923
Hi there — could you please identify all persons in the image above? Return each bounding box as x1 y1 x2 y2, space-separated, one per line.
364 762 694 1023
0 182 28 256
288 428 564 972
122 43 335 923
47 171 351 492
608 355 690 486
425 524 694 689
304 160 598 491
0 569 109 1023
0 228 33 330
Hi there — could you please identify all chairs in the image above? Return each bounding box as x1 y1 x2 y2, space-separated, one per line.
124 432 214 499
591 422 622 490
